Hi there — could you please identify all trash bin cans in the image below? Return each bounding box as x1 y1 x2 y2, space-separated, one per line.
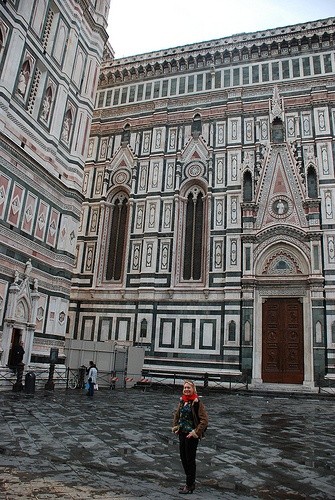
24 372 36 394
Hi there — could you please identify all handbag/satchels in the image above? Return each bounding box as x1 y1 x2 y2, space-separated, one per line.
85 382 90 389
88 377 92 383
94 384 98 390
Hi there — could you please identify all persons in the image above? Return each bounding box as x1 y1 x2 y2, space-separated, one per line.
25 258 32 276
34 276 39 293
86 361 97 396
171 381 209 494
16 341 25 372
43 378 55 395
13 269 20 284
25 371 35 394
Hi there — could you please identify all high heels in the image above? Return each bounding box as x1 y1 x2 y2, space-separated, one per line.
178 486 194 494
